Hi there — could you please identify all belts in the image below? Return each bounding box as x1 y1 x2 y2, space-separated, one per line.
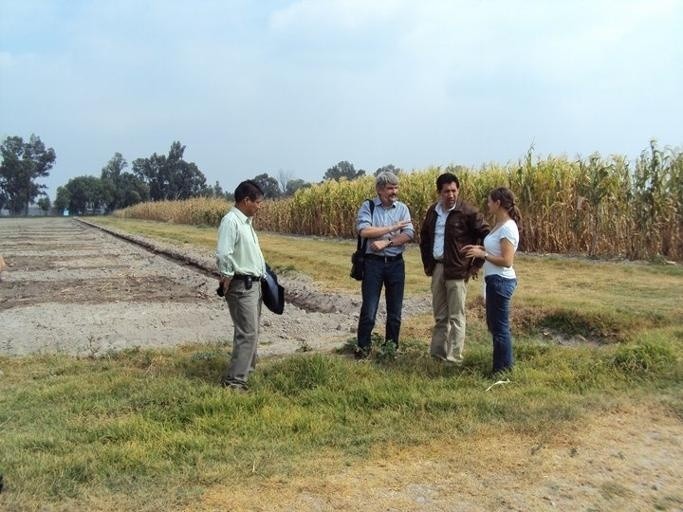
367 253 403 261
233 274 260 281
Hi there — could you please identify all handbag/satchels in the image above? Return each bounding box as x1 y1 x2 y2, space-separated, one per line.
350 251 366 281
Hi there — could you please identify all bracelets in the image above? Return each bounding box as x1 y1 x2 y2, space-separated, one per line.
485 253 489 260
388 238 393 248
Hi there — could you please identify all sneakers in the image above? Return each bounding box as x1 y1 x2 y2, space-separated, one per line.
356 348 371 360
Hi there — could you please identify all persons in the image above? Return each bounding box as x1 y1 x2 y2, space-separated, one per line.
420 174 491 367
459 187 521 380
353 171 415 359
216 180 267 391
